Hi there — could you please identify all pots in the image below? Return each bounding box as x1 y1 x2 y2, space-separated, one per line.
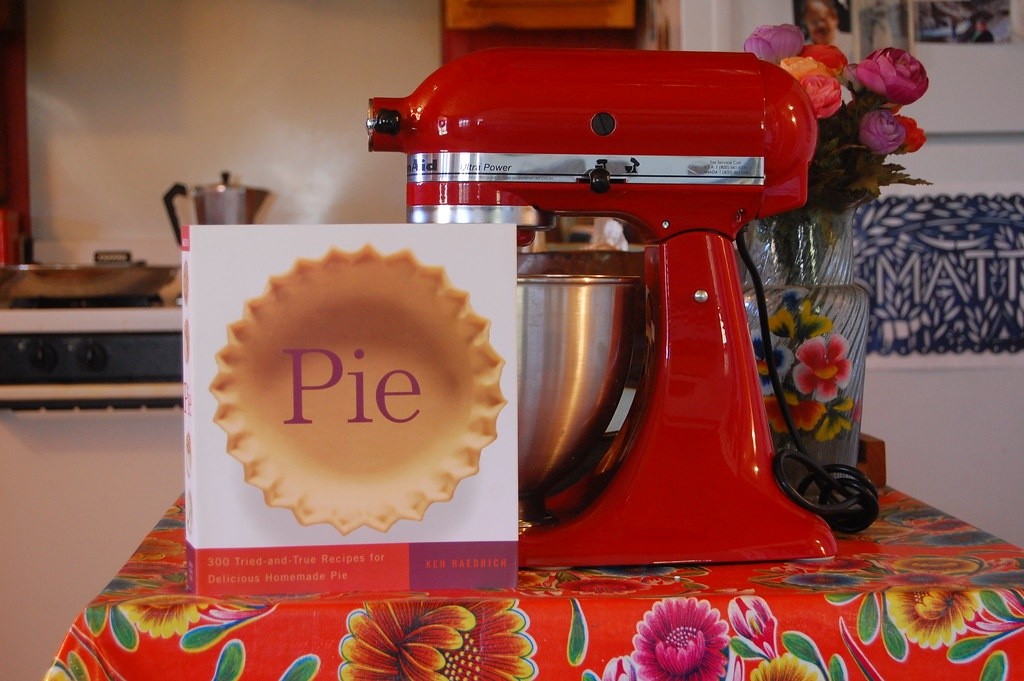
0 252 182 295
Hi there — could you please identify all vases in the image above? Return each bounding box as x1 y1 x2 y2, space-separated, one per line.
740 214 871 497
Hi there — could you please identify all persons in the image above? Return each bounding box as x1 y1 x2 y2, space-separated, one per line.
800 0 852 63
932 1 1013 44
869 0 894 51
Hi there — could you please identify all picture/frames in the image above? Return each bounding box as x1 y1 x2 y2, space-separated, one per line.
850 0 916 61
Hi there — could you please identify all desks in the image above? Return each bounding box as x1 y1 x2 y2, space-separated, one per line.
44 490 1024 679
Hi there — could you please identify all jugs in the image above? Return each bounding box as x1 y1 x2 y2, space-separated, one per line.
163 171 267 245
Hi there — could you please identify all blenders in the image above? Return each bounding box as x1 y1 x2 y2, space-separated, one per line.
366 46 837 570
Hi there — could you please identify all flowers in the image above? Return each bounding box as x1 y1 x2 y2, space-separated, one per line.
743 23 935 210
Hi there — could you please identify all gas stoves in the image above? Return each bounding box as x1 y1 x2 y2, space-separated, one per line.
0 304 186 413
